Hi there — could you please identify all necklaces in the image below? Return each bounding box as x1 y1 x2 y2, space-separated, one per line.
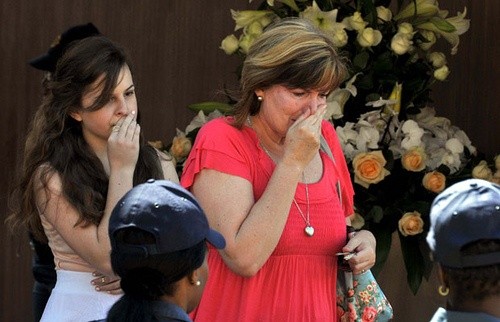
247 115 314 237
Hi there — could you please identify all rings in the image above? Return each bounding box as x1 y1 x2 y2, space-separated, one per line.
362 269 365 273
101 277 105 283
112 125 120 131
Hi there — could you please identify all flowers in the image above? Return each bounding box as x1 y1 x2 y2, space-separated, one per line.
148 109 219 173
331 81 500 296
190 1 469 126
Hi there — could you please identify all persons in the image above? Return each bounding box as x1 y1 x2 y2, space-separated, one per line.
426 178 500 322
180 16 376 322
6 21 181 322
87 179 226 322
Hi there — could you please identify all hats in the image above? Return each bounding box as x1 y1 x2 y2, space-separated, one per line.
107 178 227 259
425 177 500 269
28 20 103 71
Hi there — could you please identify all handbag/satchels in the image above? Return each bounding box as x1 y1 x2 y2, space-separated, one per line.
320 123 394 322
